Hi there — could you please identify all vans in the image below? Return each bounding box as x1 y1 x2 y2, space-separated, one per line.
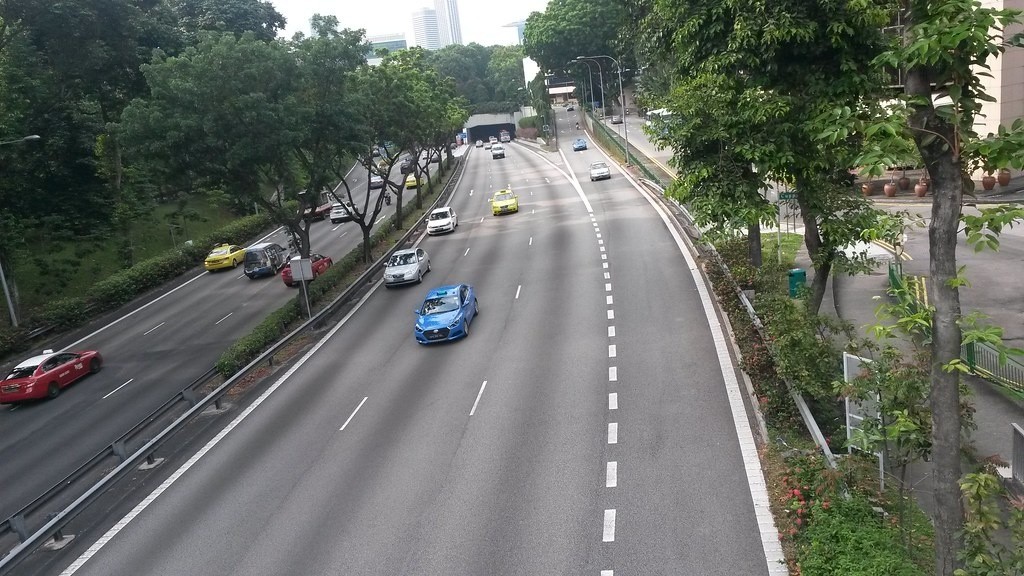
296 188 332 223
242 242 291 279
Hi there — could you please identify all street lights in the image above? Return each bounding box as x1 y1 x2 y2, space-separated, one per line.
576 55 630 166
567 59 606 137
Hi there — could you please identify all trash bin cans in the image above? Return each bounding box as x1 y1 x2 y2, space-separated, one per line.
789 268 807 299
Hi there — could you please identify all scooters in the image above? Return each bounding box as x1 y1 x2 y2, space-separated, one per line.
576 124 579 130
385 194 391 206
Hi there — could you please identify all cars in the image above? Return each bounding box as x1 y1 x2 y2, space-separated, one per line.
573 139 587 151
425 206 458 236
611 115 622 124
405 173 424 189
0 348 103 404
475 130 510 159
370 176 384 189
371 141 458 177
562 102 575 111
282 252 334 287
489 189 518 216
382 248 432 288
204 243 249 272
413 283 479 346
589 161 611 182
330 201 358 224
